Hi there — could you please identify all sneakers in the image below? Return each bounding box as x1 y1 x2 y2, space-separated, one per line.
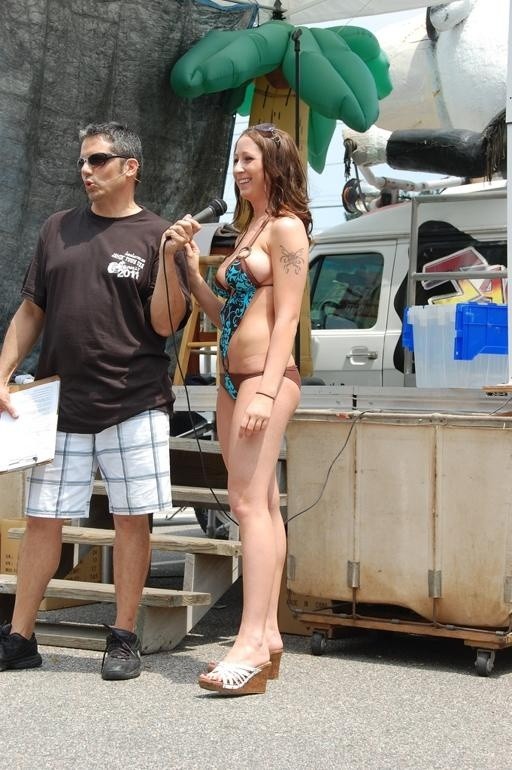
100 623 142 680
0 632 42 673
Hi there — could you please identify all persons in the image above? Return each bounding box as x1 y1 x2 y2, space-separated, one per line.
174 123 318 697
1 118 203 682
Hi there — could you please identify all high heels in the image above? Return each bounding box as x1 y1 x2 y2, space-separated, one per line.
206 648 282 680
199 660 272 695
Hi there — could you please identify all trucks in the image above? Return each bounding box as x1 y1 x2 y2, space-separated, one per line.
195 174 507 544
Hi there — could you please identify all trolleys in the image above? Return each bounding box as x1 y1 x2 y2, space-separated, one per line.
288 191 511 678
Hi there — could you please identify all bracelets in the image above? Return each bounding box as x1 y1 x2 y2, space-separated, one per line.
252 391 278 403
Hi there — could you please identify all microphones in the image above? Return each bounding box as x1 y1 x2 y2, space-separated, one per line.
166 199 227 240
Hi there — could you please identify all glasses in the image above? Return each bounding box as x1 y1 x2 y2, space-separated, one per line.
243 122 280 150
76 152 127 170
